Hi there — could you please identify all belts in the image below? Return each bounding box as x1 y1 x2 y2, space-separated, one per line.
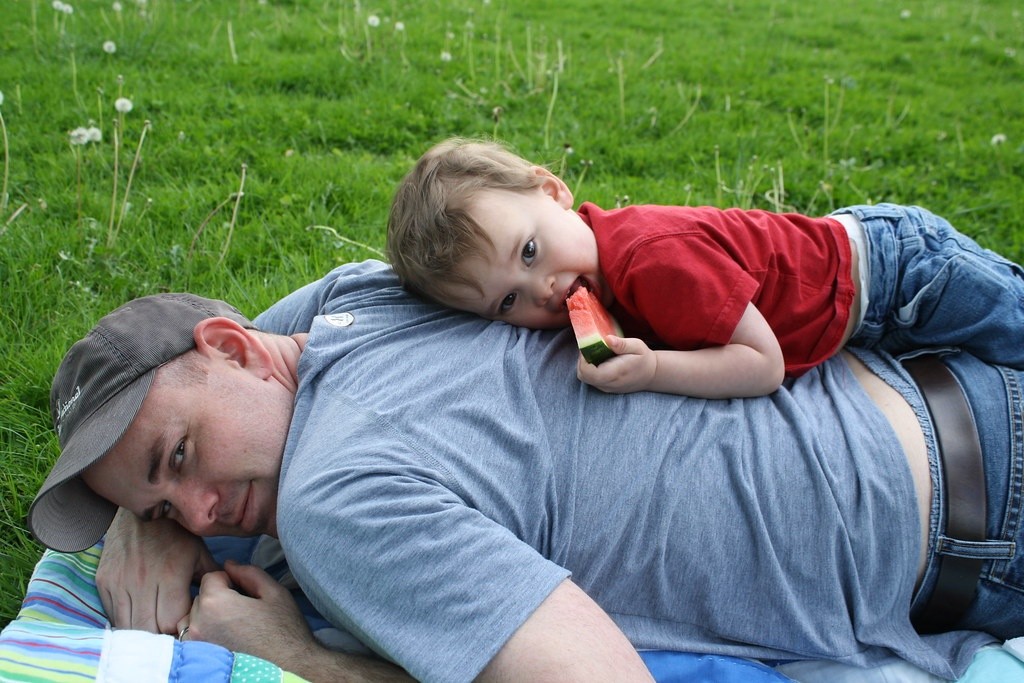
903 351 984 634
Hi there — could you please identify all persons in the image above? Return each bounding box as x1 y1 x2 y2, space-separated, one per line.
30 258 1024 682
388 138 1024 398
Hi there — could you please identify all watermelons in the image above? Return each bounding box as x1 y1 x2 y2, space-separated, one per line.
566 286 624 365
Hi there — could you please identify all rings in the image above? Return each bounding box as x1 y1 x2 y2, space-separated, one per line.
179 627 189 641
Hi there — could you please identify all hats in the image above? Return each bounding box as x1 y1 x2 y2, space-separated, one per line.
30 293 263 554
